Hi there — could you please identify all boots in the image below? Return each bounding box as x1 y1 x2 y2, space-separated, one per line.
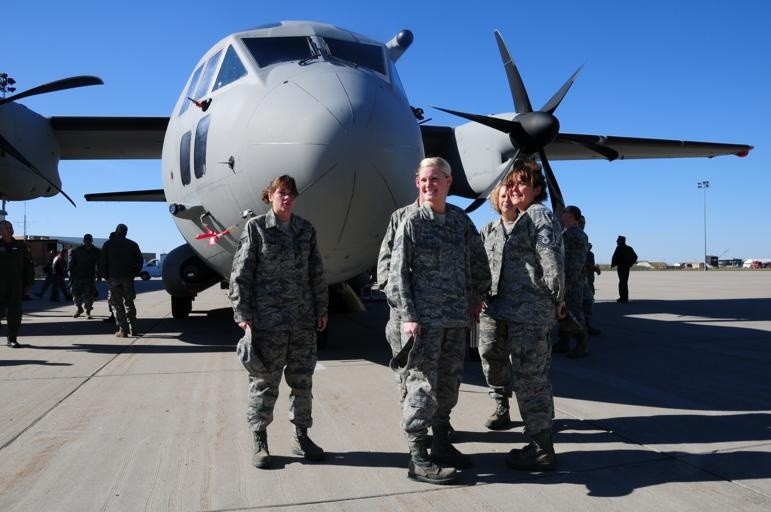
115 328 145 338
7 336 21 348
73 306 92 320
407 442 465 485
485 400 511 428
290 425 325 460
509 429 557 473
251 430 271 470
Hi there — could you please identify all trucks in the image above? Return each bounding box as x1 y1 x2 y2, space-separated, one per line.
139 257 162 280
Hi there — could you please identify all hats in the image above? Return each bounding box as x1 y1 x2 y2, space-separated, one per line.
237 324 268 376
390 333 426 375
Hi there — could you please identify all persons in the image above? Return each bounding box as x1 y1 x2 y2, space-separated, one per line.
374 154 599 487
611 236 639 299
1 219 143 348
228 174 329 468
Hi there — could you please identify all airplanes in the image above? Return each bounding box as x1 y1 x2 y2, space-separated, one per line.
0 21 754 321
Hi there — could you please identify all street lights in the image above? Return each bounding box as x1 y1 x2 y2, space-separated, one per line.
0 72 15 210
696 180 709 271
